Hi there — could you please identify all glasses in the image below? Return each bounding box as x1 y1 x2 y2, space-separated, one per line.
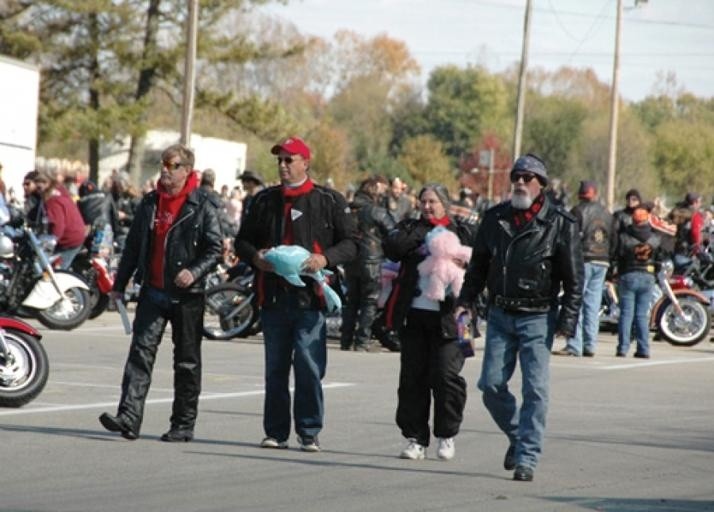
512 173 536 183
158 158 189 170
278 157 302 164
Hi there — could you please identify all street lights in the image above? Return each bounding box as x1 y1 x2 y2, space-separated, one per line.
469 167 510 200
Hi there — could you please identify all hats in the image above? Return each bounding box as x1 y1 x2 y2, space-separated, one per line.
237 170 262 184
460 187 479 198
632 208 648 223
511 155 549 187
578 180 597 198
271 136 310 160
626 188 641 204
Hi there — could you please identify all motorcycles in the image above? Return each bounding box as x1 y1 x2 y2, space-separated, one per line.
1 315 50 408
0 195 145 330
554 234 714 347
197 212 402 353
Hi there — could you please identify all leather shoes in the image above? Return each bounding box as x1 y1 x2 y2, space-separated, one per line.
514 466 533 481
161 431 194 441
100 413 137 439
504 444 517 469
550 349 576 355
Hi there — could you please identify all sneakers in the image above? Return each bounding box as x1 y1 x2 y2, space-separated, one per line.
262 436 288 448
438 437 456 460
298 437 320 451
401 438 426 460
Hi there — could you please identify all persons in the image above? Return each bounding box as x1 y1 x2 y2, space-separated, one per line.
1 170 714 357
234 137 361 451
454 153 585 481
99 145 224 441
381 184 478 460
